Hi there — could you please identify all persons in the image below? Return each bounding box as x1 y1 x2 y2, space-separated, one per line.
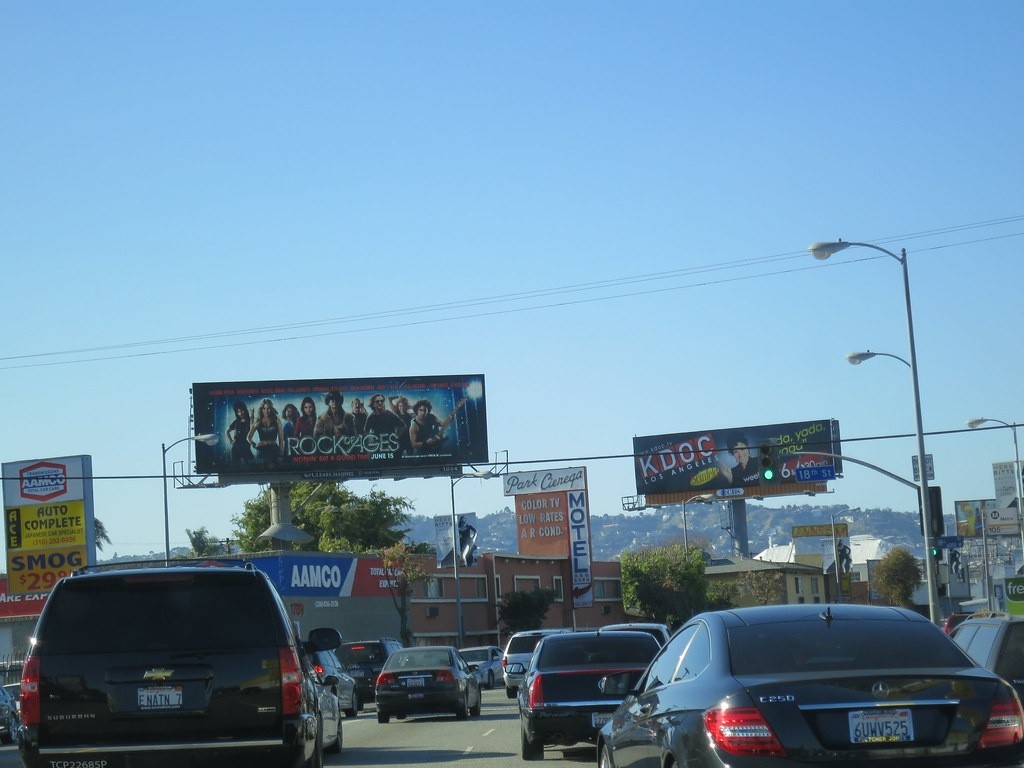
225 393 456 458
719 432 762 488
442 516 478 568
826 539 852 574
950 548 961 574
975 501 987 518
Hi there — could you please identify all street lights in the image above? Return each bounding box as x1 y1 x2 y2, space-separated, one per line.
161 433 219 569
807 239 940 630
964 417 1024 544
828 504 862 603
448 471 493 645
681 495 718 564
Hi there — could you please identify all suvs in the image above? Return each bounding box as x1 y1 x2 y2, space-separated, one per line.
15 560 342 767
334 636 407 712
944 610 1024 710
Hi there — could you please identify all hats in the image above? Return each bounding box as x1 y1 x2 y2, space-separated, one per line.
325 391 343 405
233 401 247 413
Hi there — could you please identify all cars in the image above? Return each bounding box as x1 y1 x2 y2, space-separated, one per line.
501 629 571 699
504 630 663 763
588 623 672 663
376 646 482 725
0 686 20 744
301 639 360 717
597 603 1024 768
454 646 505 690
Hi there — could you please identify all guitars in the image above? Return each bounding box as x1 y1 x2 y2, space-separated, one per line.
422 397 469 450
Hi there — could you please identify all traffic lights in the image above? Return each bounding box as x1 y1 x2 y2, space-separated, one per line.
932 548 941 560
759 442 782 487
